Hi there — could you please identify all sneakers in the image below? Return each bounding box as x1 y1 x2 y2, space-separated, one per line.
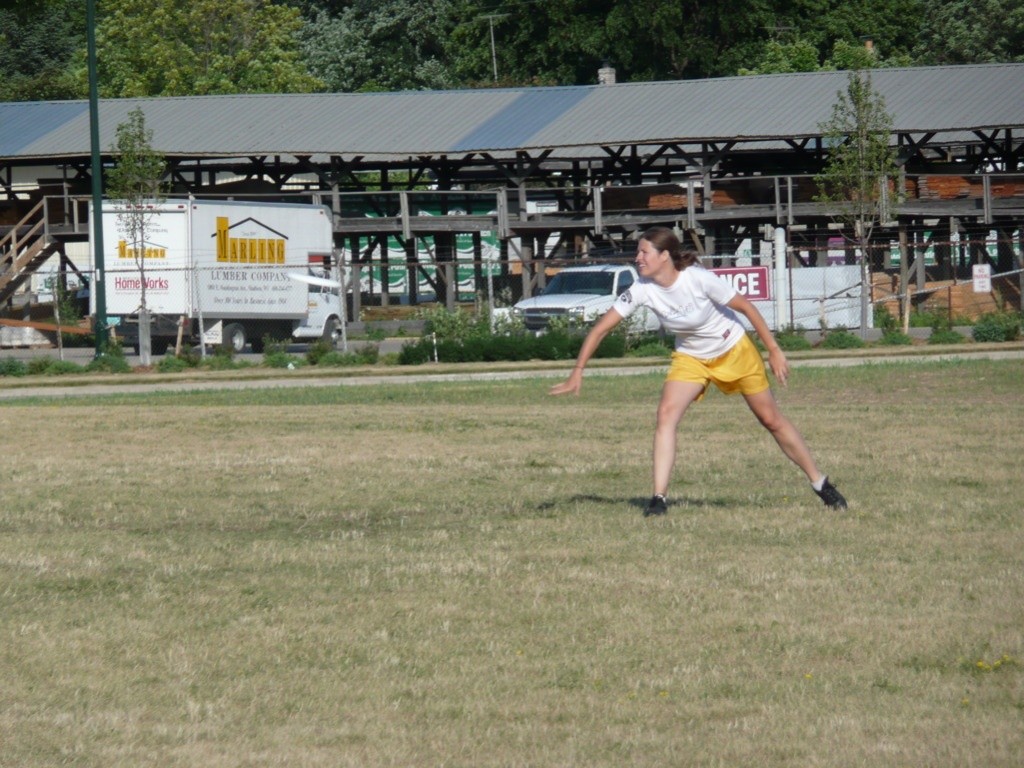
814 477 847 511
644 496 669 518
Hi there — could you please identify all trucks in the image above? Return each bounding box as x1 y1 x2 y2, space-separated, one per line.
89 198 348 354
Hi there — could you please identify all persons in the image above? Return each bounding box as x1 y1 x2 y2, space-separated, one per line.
545 228 849 517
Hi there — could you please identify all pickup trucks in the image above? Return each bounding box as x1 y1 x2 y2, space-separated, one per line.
510 264 668 345
